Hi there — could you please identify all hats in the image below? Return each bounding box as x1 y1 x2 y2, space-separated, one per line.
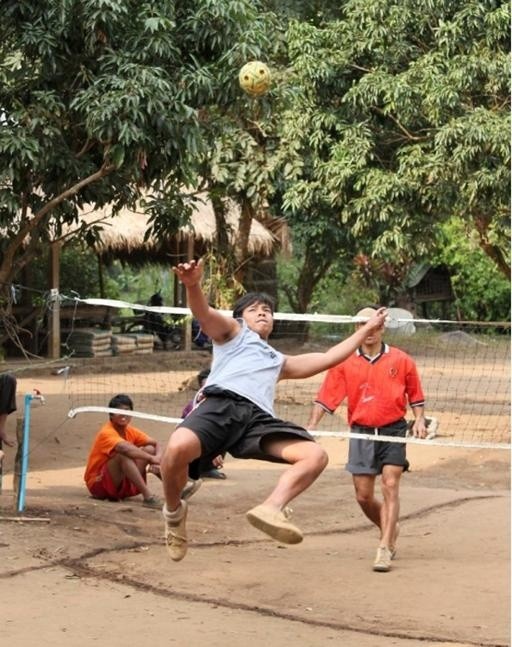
356 308 377 321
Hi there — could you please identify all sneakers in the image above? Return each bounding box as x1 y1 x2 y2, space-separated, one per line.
389 523 399 559
142 494 166 510
180 478 202 500
163 500 188 561
246 504 302 544
201 469 226 480
374 544 392 571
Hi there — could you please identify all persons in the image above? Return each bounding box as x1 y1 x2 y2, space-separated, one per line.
301 306 429 572
181 369 226 480
160 257 387 561
0 374 17 468
84 394 203 510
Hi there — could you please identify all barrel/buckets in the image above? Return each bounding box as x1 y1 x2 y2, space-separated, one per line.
384 308 418 339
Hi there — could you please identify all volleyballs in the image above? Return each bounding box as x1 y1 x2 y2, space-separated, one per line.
239 60 271 94
